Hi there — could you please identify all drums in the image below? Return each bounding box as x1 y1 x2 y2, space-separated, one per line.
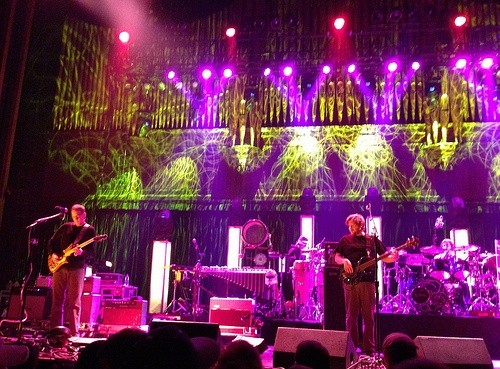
474 273 496 291
398 271 426 295
292 260 316 307
443 282 460 299
242 245 269 271
242 219 269 247
452 260 471 279
406 276 455 314
315 263 324 313
425 258 451 280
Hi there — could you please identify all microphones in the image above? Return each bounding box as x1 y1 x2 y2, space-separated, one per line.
54 206 69 213
242 312 249 321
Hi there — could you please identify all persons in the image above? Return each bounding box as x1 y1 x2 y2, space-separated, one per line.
434 239 456 271
0 321 337 369
383 332 434 369
39 204 96 336
334 213 399 359
286 237 308 272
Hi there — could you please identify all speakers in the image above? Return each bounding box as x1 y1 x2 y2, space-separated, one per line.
272 327 357 369
413 336 493 369
101 301 148 327
151 319 223 345
209 298 256 328
8 294 50 321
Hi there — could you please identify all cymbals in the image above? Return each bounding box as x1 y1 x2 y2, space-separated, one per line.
479 253 492 257
420 246 445 254
384 246 407 256
397 253 430 266
451 246 478 251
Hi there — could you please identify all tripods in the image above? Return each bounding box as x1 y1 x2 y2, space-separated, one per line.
282 252 500 322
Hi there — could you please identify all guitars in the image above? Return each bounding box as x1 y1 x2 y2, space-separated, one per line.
48 234 108 273
339 235 420 284
